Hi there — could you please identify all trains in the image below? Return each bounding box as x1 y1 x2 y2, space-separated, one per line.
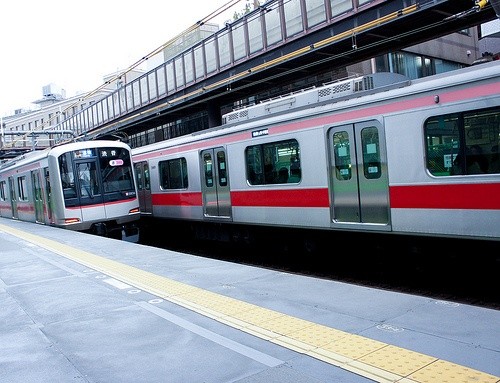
128 57 500 244
0 138 142 232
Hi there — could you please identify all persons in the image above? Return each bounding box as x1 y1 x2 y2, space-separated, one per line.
449 144 500 174
334 150 380 181
247 155 300 184
108 166 124 181
